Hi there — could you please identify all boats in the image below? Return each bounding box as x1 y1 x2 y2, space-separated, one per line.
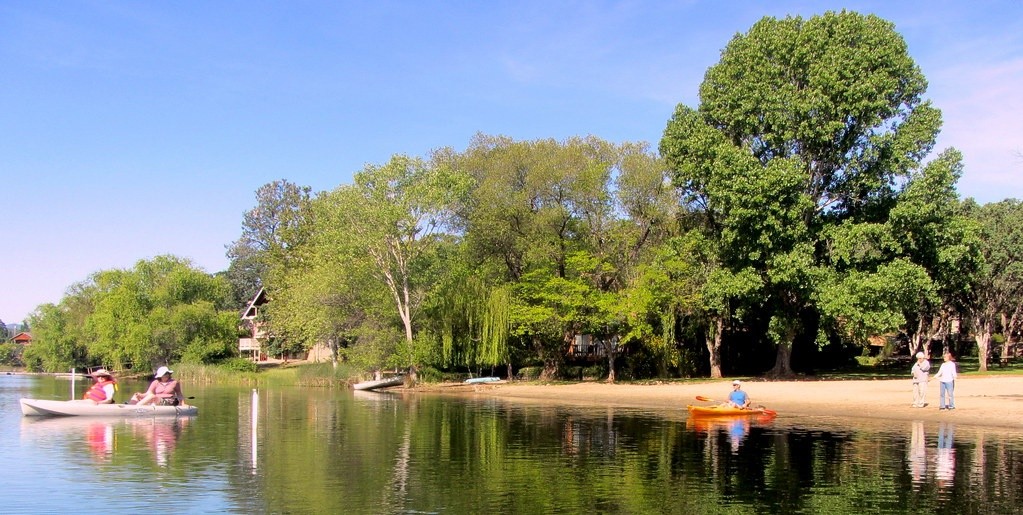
465 377 500 383
686 403 777 418
352 376 404 390
685 415 777 432
19 396 200 417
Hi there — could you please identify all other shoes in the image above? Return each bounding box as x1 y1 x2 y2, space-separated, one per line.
940 408 946 411
909 406 923 408
949 407 955 410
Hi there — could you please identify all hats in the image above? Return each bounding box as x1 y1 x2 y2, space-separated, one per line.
155 366 173 378
732 380 740 385
90 369 111 376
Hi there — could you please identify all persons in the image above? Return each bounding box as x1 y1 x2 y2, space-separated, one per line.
933 351 956 409
911 352 930 408
128 367 189 407
82 369 117 406
718 380 751 411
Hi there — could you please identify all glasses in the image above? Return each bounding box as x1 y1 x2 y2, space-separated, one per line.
733 385 737 386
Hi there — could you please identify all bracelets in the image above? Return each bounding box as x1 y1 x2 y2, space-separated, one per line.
96 401 98 405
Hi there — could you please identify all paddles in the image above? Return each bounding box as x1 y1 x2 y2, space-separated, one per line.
696 395 768 414
